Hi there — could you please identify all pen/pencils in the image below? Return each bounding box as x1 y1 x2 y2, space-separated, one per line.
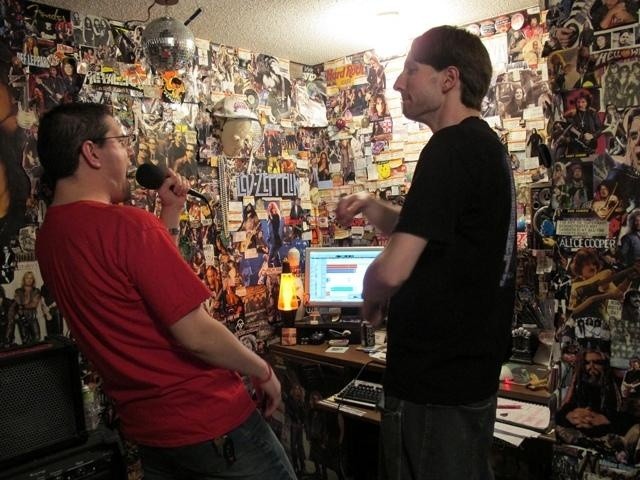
497 403 521 408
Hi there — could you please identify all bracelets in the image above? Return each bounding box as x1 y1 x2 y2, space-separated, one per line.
253 362 272 384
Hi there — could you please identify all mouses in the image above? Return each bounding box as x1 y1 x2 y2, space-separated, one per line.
499 365 514 382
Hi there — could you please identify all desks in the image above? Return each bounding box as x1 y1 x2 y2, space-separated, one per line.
270 344 554 480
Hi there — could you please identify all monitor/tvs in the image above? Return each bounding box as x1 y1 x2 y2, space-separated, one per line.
305 247 385 321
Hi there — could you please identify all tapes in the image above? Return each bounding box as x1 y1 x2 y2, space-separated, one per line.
342 329 351 337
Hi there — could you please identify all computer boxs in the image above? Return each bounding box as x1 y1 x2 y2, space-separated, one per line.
294 316 376 347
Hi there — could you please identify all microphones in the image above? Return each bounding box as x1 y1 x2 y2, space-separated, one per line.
135 163 210 204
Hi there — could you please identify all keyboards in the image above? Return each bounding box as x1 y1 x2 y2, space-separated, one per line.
334 379 383 410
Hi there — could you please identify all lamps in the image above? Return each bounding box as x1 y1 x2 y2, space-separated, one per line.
140 1 197 73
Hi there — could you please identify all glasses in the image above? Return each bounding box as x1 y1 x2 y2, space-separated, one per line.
89 134 136 147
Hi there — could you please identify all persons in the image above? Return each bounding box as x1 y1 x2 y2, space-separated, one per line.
33 102 298 479
471 0 640 468
335 25 514 479
0 0 411 352
